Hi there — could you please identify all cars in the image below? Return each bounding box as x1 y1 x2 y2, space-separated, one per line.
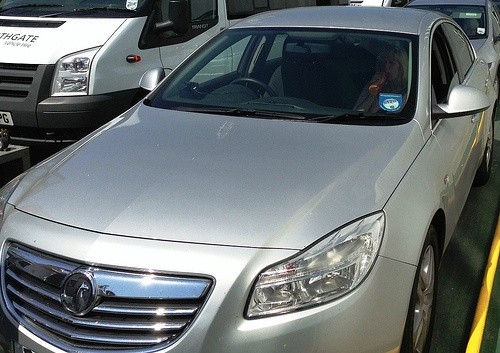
0 4 499 353
402 0 500 95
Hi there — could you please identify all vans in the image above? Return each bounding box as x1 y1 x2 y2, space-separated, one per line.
0 0 351 148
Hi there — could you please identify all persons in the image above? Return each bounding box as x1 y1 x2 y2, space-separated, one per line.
353 50 408 114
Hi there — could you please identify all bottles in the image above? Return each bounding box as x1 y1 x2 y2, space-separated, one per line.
368 69 388 96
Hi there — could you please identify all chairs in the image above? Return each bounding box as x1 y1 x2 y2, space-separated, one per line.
264 35 337 96
